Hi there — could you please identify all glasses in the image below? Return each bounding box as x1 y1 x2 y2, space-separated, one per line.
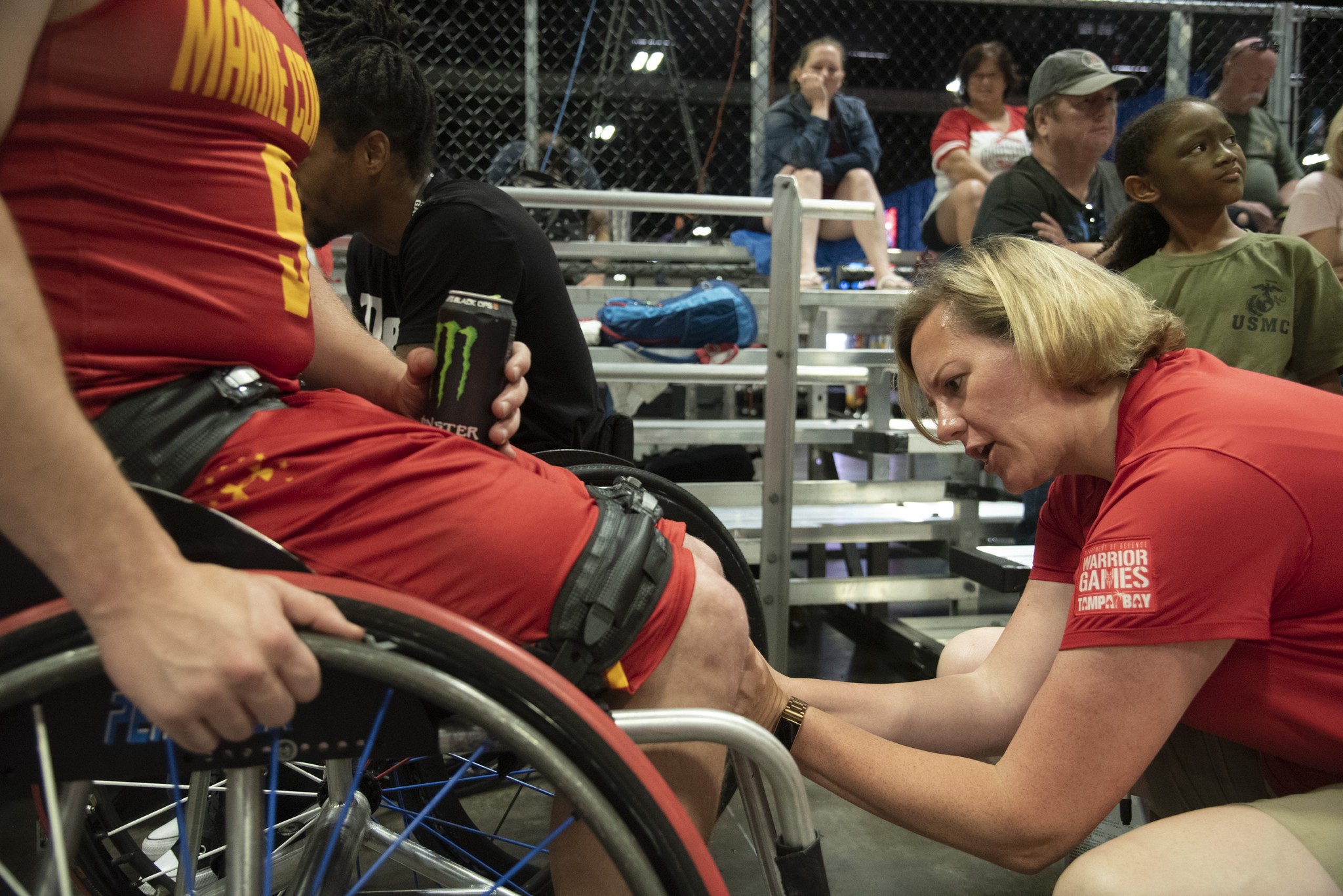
1232 40 1281 60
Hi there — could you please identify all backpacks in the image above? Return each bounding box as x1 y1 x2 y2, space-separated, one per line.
596 281 759 364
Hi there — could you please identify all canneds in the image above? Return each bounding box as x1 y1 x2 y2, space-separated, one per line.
420 289 519 448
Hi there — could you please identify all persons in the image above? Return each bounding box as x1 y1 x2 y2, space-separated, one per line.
0 0 1343 896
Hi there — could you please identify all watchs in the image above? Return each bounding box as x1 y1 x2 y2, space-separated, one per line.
773 696 808 751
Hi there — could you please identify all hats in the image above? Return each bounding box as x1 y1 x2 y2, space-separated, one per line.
1028 49 1141 107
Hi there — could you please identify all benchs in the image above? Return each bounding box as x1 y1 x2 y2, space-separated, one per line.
491 180 1024 678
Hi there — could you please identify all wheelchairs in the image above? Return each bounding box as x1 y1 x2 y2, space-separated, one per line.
0 449 838 896
